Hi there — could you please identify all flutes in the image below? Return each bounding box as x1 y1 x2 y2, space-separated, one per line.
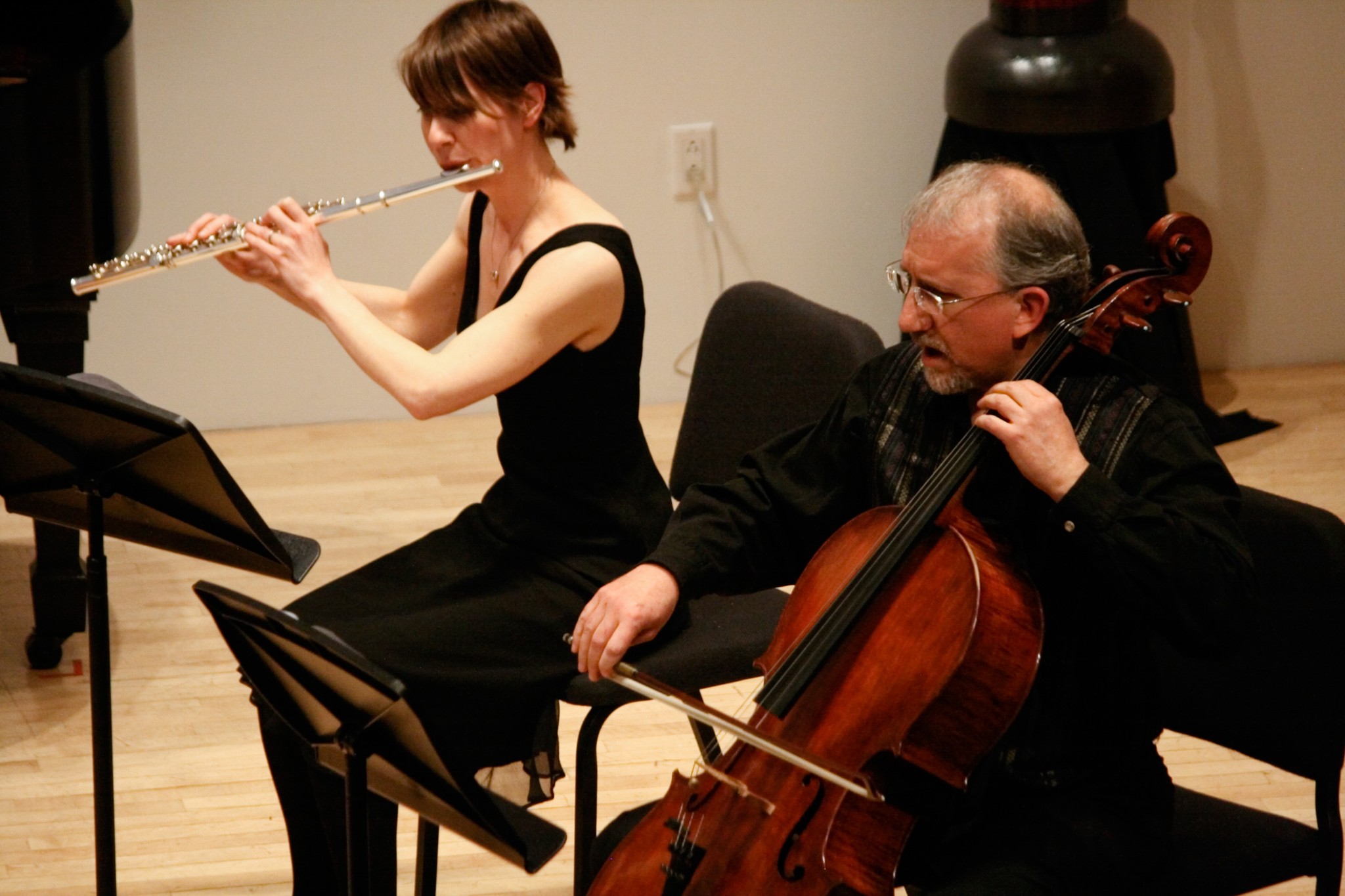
70 158 504 295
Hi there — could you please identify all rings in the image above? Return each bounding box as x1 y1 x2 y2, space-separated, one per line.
265 228 281 245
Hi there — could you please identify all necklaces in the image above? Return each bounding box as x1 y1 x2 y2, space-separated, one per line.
486 157 559 285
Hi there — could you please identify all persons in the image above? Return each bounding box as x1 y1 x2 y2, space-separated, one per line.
160 1 679 896
572 158 1243 896
0 0 140 675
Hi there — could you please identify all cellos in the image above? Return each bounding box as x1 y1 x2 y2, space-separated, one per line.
586 207 1215 895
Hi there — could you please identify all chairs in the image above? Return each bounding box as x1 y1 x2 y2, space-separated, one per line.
1163 485 1345 895
412 282 887 896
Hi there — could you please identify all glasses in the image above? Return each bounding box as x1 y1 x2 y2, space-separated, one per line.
883 258 1010 315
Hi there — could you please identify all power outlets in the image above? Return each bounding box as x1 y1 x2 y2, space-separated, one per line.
668 124 714 196
